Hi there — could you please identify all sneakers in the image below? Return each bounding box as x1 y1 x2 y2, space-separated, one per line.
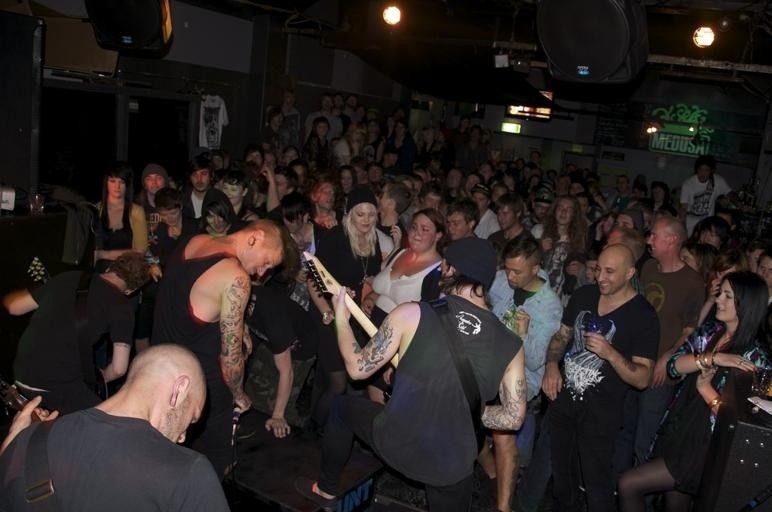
294 475 338 511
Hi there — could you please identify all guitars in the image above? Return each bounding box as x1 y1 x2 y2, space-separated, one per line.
301 251 400 367
0 379 42 423
26 256 108 403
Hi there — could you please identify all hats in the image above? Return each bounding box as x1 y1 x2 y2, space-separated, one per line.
533 189 554 204
345 187 378 212
384 146 398 155
622 207 643 231
142 163 170 184
469 183 490 197
444 236 498 286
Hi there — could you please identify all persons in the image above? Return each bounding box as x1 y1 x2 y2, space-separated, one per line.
0 88 770 512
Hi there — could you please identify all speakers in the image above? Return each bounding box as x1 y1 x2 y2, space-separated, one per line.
0 9 46 213
692 404 771 512
84 0 173 58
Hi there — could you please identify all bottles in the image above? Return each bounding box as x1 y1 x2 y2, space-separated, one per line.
586 312 598 352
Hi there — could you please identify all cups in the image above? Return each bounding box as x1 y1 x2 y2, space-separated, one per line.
29 194 45 215
749 366 772 398
